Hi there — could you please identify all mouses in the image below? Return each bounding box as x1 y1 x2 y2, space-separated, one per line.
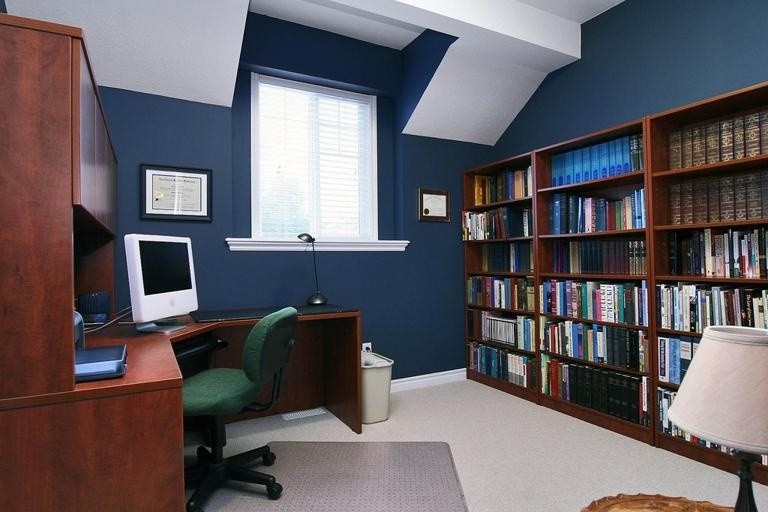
213 335 224 344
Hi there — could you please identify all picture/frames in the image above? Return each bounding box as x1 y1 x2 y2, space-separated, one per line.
141 165 213 224
419 191 451 226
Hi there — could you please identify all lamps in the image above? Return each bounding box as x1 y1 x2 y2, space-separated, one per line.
667 325 768 512
300 232 330 310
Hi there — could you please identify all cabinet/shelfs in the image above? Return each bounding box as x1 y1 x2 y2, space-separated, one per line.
0 13 120 400
463 81 768 484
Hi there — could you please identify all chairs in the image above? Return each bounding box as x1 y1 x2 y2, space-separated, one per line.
183 306 305 512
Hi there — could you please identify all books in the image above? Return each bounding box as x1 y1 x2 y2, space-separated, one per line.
655 281 767 467
550 134 644 188
668 110 767 169
466 239 535 313
466 309 536 393
539 279 650 425
669 167 767 279
546 184 648 275
461 166 533 241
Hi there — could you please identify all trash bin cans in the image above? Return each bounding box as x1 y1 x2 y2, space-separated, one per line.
360 352 395 424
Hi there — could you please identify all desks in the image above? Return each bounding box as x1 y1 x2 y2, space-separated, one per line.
0 305 364 512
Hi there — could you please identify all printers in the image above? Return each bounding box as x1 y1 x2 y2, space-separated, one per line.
74 310 127 383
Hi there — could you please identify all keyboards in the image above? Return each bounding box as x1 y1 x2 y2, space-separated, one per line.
171 338 211 358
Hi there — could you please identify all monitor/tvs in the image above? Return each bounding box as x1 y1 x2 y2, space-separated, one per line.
124 234 199 334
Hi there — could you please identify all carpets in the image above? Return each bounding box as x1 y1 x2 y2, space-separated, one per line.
201 440 469 512
